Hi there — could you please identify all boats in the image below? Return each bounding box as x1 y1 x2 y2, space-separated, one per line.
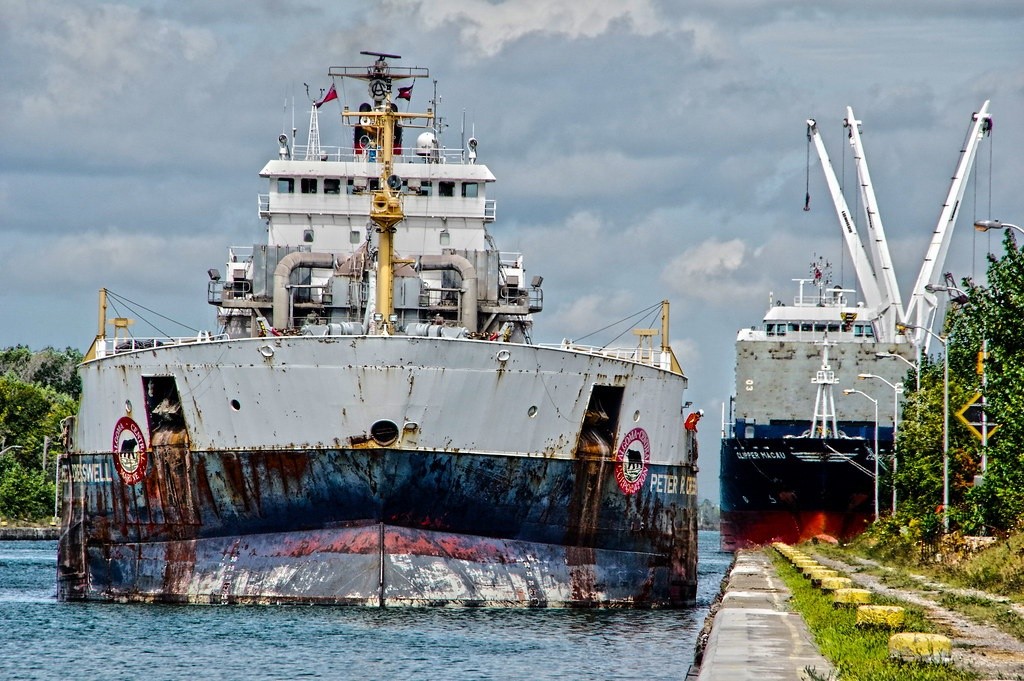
57 50 699 612
718 279 918 552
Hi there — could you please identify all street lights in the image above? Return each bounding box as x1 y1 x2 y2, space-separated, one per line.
842 219 1024 533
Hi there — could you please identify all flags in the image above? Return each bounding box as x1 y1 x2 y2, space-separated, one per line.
314 84 338 109
395 86 413 101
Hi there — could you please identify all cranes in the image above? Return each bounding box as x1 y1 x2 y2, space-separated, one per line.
803 98 993 354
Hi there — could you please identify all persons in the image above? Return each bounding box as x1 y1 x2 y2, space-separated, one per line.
685 409 704 433
369 142 382 163
